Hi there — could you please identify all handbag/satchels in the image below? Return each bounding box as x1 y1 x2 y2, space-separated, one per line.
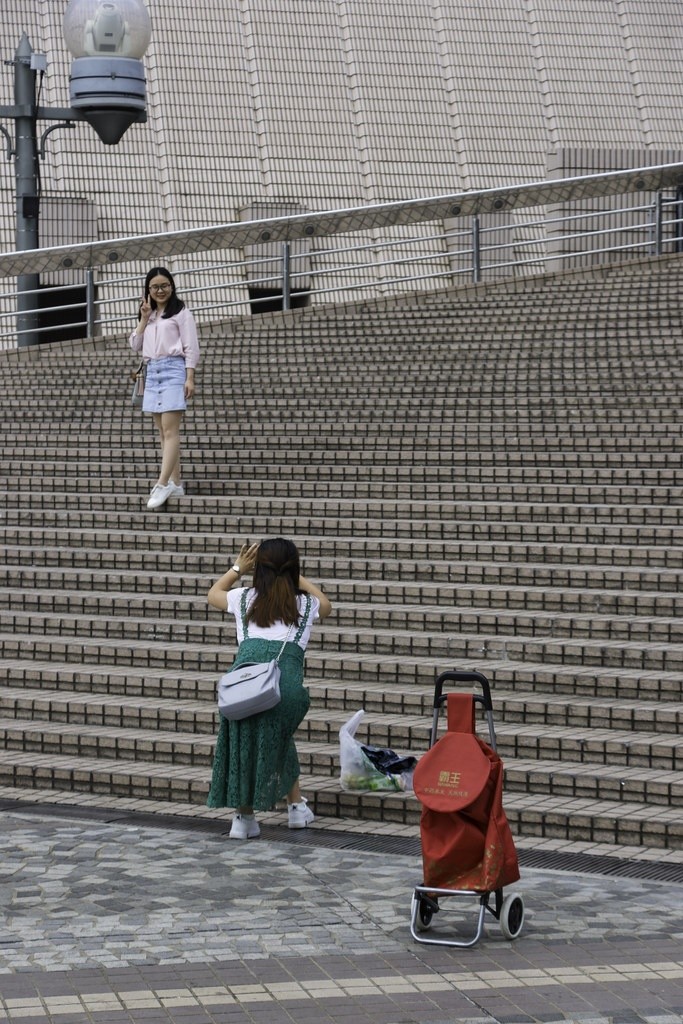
338 710 418 792
218 659 280 720
132 374 146 404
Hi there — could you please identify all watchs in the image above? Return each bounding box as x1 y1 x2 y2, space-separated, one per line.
232 563 241 577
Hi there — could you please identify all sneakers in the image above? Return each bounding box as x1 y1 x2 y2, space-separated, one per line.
168 480 184 496
147 482 172 508
230 812 260 839
288 796 315 828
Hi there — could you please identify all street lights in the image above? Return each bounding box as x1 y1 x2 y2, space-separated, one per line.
0 0 152 346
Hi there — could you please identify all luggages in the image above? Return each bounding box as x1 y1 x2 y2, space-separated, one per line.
410 670 525 945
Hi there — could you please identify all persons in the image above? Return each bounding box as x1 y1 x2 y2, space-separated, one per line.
129 266 200 509
206 538 332 839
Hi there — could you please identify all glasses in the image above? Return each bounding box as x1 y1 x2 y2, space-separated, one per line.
149 283 171 291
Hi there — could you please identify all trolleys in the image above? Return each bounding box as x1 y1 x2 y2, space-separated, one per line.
410 671 525 947
